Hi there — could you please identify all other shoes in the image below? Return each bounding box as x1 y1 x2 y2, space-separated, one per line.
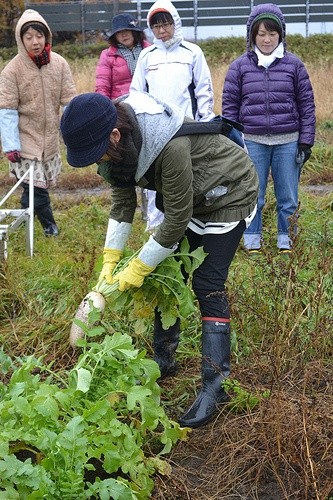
278 248 293 253
248 249 262 254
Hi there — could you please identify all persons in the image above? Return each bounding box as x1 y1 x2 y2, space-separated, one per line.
0 9 78 238
126 0 216 241
94 14 152 220
222 4 316 275
59 91 259 428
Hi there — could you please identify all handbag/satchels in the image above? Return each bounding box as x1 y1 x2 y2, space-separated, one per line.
208 115 245 152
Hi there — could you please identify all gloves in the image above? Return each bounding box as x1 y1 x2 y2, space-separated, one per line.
6 150 20 163
112 234 178 292
98 218 131 284
298 143 312 163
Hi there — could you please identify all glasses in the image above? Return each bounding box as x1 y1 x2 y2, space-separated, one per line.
150 22 174 31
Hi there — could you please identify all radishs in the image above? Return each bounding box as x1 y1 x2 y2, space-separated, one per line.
69 292 105 349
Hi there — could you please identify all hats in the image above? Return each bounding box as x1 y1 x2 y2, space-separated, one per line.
255 14 281 27
20 21 46 34
60 92 117 168
107 13 144 42
149 8 169 25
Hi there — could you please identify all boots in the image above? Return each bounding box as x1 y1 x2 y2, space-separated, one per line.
136 304 180 386
177 316 231 428
